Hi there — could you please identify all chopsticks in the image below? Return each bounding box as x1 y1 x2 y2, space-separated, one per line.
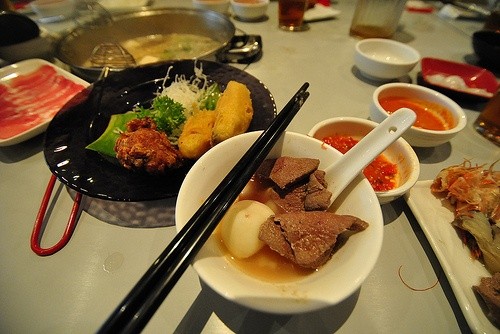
95 82 312 334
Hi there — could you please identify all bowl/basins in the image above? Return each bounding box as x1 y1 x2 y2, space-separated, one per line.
472 30 500 60
30 0 75 23
311 117 421 207
230 0 270 20
355 38 420 82
421 57 499 101
192 0 230 15
371 82 468 147
43 59 278 202
175 129 385 315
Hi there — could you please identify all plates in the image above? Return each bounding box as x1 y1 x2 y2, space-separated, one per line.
0 57 93 148
403 179 500 334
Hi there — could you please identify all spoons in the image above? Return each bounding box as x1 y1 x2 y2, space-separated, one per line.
316 107 418 211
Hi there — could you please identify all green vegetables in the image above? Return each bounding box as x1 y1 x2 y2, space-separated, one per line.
134 82 220 132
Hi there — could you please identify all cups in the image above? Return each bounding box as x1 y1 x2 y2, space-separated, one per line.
278 0 306 31
350 0 408 39
473 88 500 147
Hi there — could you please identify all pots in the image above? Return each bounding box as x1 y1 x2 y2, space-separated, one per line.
52 6 263 83
